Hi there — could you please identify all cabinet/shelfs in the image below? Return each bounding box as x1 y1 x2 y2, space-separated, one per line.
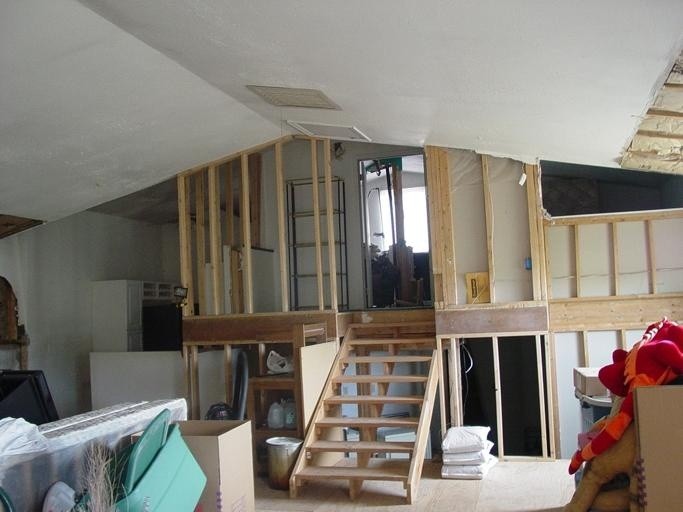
283 174 354 313
91 280 175 352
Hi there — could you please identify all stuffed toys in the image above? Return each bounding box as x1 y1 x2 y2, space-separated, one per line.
551 317 683 512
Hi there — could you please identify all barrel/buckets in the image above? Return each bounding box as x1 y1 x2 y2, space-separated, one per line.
266 436 303 489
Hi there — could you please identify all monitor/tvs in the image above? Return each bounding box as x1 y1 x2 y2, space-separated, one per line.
0 370 60 425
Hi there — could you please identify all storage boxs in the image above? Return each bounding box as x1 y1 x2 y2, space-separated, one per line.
131 414 256 512
